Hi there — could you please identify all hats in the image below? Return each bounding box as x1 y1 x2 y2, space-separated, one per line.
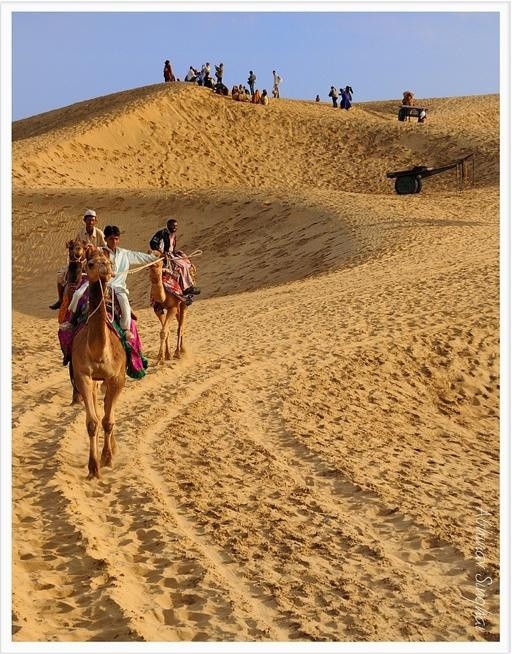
85 210 96 216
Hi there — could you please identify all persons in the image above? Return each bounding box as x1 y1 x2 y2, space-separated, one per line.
402 93 415 123
164 60 281 105
316 95 320 102
49 210 108 310
329 86 353 111
150 219 200 296
59 226 168 341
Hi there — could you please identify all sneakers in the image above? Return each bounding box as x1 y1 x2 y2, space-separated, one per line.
59 322 73 332
124 331 135 340
49 302 61 310
